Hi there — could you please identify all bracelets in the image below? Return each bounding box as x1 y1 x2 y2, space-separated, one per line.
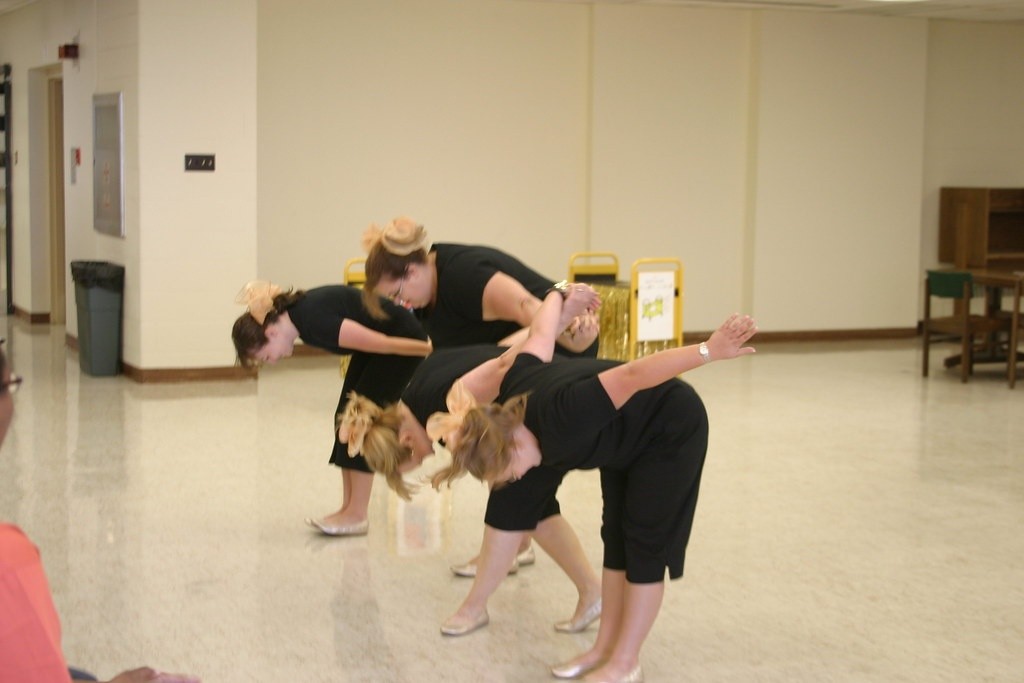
550 283 569 300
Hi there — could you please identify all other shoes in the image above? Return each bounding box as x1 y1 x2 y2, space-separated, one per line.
304 518 369 536
552 664 585 678
618 664 643 683
440 612 489 635
450 557 517 576
516 547 535 565
554 597 602 633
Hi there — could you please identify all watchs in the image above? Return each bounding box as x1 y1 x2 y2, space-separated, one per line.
699 342 710 363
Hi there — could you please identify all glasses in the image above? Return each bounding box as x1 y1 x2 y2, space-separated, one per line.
389 265 407 306
0 373 21 396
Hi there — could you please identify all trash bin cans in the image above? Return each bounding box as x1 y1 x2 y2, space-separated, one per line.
70 258 125 377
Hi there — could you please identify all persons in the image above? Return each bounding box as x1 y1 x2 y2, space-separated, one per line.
0 344 202 683
362 220 600 576
339 284 600 635
233 284 433 534
432 280 759 683
